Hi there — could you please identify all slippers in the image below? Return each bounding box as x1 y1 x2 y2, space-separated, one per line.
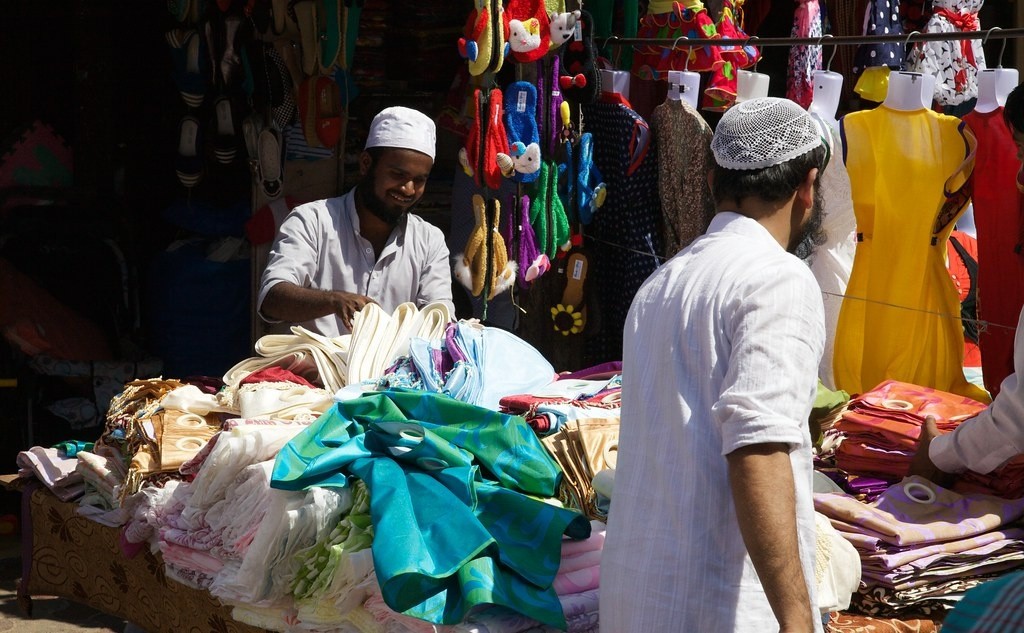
453 195 487 298
482 198 526 316
528 165 547 257
491 1 509 74
459 0 493 78
577 131 607 228
547 158 571 259
543 0 582 50
478 89 510 190
549 251 587 336
506 81 543 182
502 0 551 64
459 89 483 189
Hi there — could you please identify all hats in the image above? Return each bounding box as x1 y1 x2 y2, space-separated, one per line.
709 96 823 170
365 105 436 164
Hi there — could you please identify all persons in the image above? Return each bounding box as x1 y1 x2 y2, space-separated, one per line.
649 70 714 260
583 68 660 364
808 71 856 392
256 106 456 333
737 70 770 101
922 82 1024 486
599 97 827 633
960 68 1024 393
832 71 991 406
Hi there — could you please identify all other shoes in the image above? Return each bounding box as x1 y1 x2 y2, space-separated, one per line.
163 0 363 200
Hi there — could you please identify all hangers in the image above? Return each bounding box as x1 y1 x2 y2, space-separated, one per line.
595 25 1022 164
